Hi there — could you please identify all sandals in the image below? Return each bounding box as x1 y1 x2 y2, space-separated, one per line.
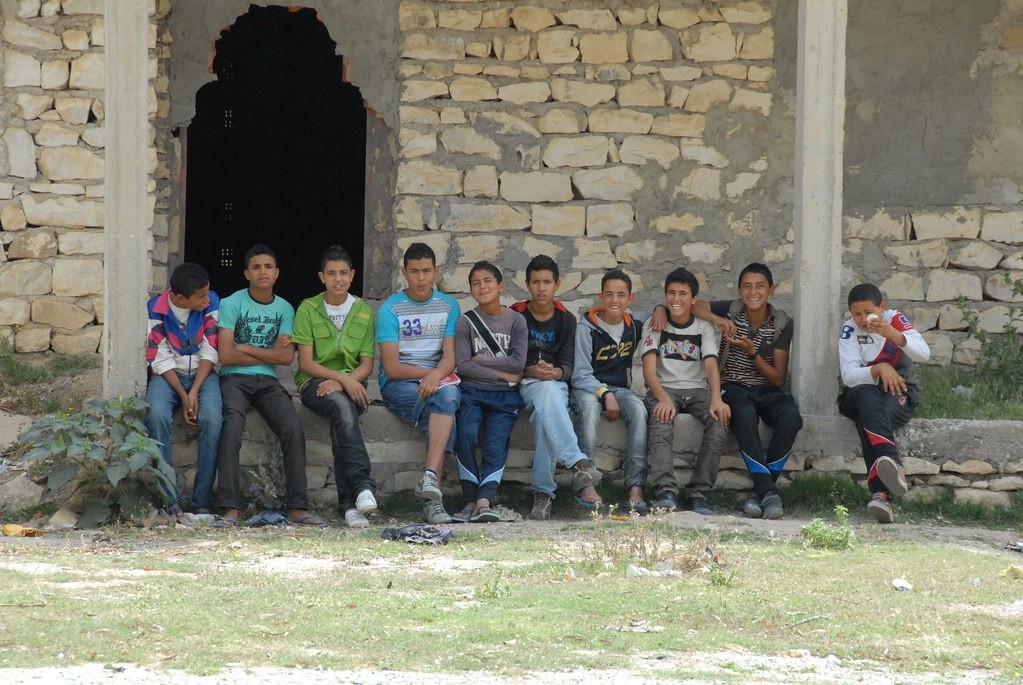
168 506 182 518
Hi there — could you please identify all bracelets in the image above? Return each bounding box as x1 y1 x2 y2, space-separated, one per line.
747 351 758 360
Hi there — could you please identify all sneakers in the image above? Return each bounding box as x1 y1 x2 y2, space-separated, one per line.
355 490 378 513
868 492 893 523
422 500 452 524
877 456 908 495
760 491 783 518
657 491 678 509
687 497 713 515
572 459 603 482
345 508 370 528
413 473 442 500
530 491 553 520
743 492 762 518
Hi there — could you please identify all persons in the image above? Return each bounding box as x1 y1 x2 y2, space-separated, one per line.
509 255 604 518
567 270 648 512
838 284 930 523
639 267 731 515
291 251 375 528
214 245 328 529
147 262 221 516
454 262 529 522
690 263 803 519
374 243 462 523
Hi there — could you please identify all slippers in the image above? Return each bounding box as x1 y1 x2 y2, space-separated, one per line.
452 509 473 521
470 506 500 522
574 495 605 507
295 515 328 527
215 517 236 528
627 500 648 510
